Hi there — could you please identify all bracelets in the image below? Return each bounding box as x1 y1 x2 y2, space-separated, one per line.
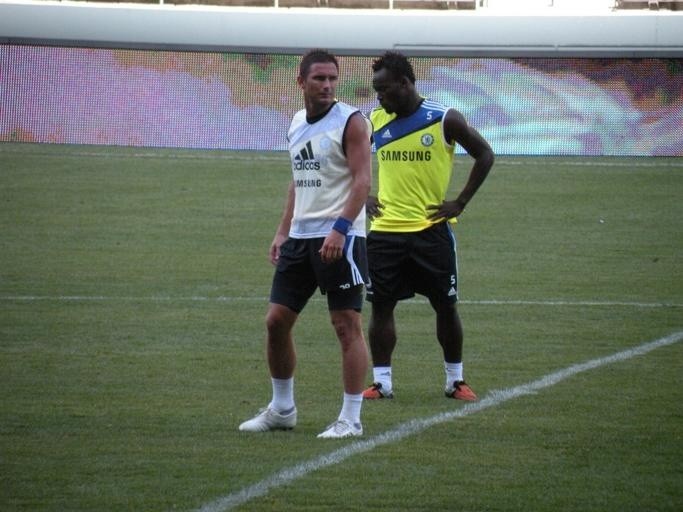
332 215 354 235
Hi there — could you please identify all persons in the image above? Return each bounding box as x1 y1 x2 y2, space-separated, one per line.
236 50 372 445
364 51 496 403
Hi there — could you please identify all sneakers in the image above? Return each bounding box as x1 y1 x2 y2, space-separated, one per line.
238 406 298 433
318 420 362 439
362 382 394 400
444 382 476 401
